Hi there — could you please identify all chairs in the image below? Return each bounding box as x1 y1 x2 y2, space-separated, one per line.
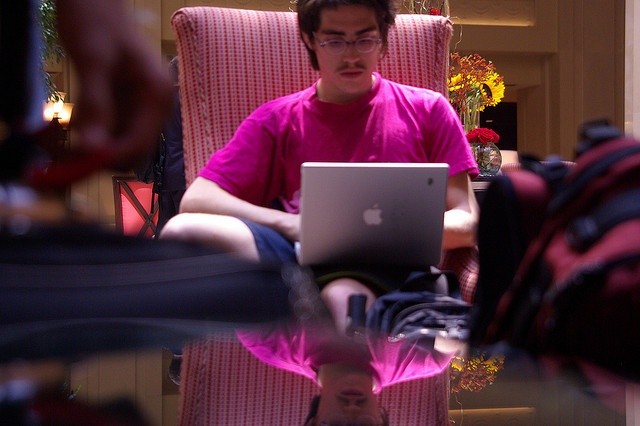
110 174 160 239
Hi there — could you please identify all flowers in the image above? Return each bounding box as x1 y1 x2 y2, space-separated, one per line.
445 352 506 390
465 127 500 173
448 51 505 130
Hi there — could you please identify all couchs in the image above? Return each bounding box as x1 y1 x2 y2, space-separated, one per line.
179 335 452 425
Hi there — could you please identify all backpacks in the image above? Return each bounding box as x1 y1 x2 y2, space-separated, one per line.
135 116 183 213
477 118 640 381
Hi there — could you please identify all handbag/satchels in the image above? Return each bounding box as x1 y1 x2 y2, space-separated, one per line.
367 268 488 346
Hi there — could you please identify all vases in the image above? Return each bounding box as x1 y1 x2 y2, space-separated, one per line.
460 96 481 135
468 143 503 175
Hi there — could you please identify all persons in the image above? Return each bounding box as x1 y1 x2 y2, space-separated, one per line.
230 321 466 424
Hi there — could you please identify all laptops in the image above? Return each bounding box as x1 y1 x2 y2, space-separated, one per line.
294 162 450 267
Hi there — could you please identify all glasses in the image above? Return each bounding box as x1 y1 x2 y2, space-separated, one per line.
312 31 383 56
320 415 375 425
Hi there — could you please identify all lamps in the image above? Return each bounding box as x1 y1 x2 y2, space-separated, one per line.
44 91 77 131
